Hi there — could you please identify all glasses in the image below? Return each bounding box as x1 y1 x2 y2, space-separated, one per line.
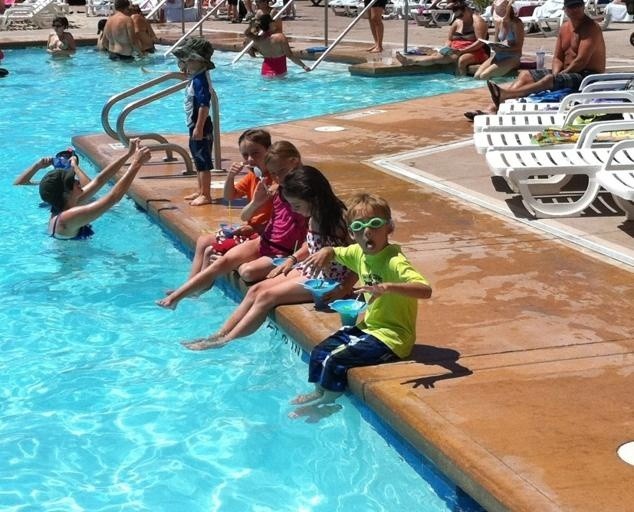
51 24 66 28
49 157 73 170
454 13 464 19
348 217 389 232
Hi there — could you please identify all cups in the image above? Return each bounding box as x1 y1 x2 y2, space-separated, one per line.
536 51 545 69
303 279 340 308
328 298 367 327
272 258 287 267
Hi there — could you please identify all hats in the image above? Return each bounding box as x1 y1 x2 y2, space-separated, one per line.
172 35 216 70
39 168 76 205
492 0 512 23
260 14 276 23
564 0 584 6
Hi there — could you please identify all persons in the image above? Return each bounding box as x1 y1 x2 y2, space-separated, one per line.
166 130 272 297
228 0 311 79
15 150 88 186
395 0 489 76
474 1 524 79
46 19 75 58
156 141 306 311
364 1 387 54
288 194 433 423
465 1 605 119
95 0 156 63
35 139 153 243
178 165 353 352
175 37 214 205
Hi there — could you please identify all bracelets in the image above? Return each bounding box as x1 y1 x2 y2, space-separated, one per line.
287 256 297 262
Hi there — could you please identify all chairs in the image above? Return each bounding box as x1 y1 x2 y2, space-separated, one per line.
0 0 168 29
327 0 602 36
497 80 634 112
472 105 634 155
473 92 634 132
485 122 634 219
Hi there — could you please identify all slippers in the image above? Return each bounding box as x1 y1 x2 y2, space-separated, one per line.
404 49 427 56
463 110 487 120
486 79 501 107
